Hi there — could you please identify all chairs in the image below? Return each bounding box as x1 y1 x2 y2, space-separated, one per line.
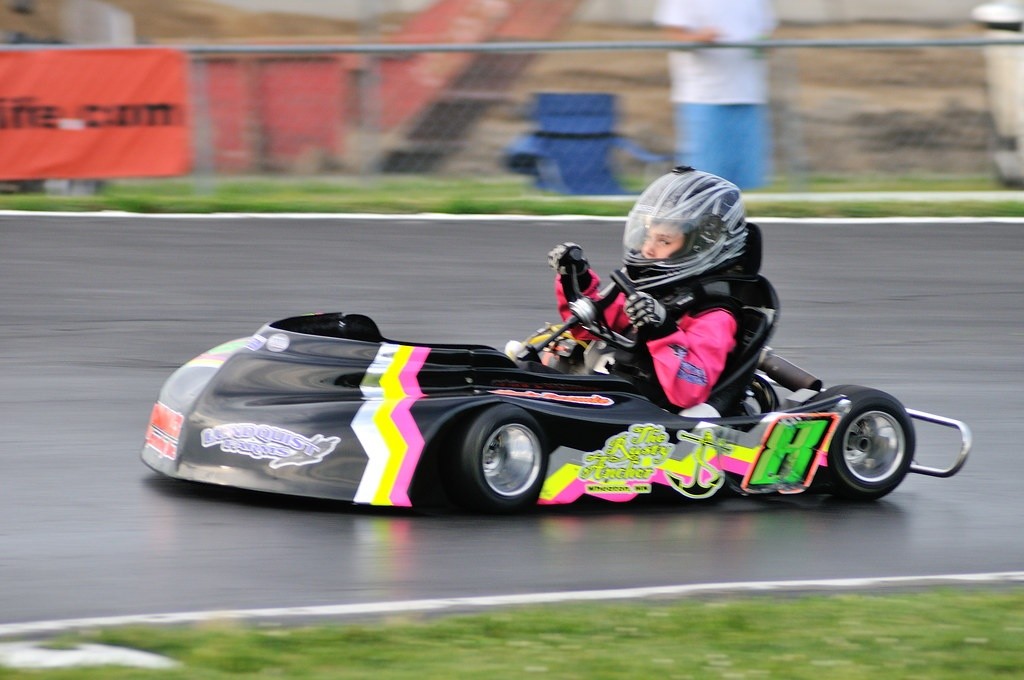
670 221 781 418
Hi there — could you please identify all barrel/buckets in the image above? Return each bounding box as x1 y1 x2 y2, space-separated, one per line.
531 92 617 195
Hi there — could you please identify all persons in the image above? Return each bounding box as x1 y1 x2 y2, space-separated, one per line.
539 164 748 425
656 0 778 190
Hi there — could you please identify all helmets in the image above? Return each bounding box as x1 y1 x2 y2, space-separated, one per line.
621 166 749 291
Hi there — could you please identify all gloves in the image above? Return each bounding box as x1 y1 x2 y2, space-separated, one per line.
548 242 592 292
623 292 676 338
